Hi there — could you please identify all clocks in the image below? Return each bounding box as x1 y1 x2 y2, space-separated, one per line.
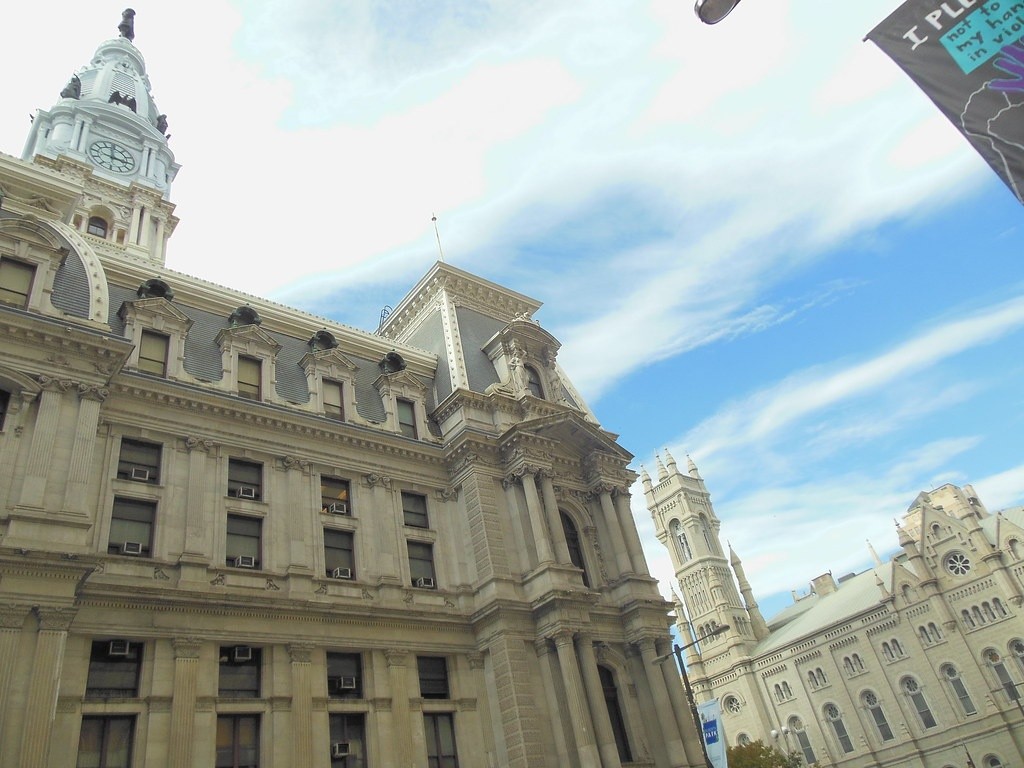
89 141 135 173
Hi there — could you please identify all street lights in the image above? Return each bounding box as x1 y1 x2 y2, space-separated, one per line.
648 622 731 768
